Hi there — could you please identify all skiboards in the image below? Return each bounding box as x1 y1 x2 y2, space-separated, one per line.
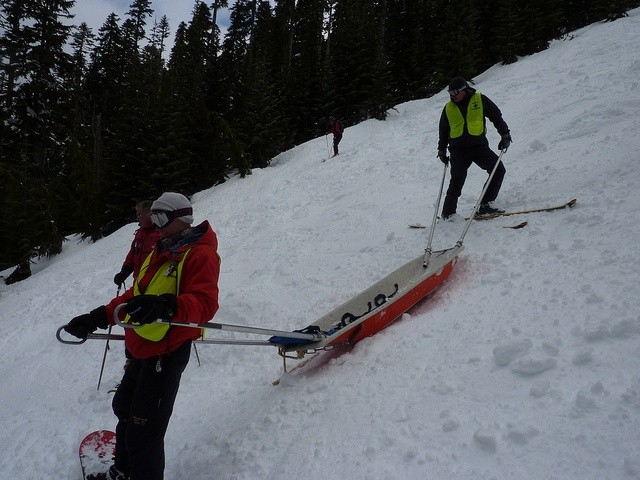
408 198 577 231
322 154 340 162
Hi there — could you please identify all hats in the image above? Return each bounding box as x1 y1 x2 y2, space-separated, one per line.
150 192 195 225
449 76 466 90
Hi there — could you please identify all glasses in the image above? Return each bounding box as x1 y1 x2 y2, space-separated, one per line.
147 207 193 229
447 85 466 96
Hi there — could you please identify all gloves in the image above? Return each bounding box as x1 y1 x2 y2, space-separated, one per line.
123 293 179 324
65 304 108 339
114 266 134 286
498 130 513 150
436 147 450 165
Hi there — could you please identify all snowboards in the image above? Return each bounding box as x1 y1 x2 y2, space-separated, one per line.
79 430 116 480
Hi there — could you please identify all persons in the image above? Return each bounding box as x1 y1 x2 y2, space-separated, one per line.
65 192 221 480
325 116 344 157
438 77 511 218
114 201 163 284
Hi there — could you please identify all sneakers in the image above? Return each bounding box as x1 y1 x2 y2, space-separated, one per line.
479 202 498 214
86 465 129 480
